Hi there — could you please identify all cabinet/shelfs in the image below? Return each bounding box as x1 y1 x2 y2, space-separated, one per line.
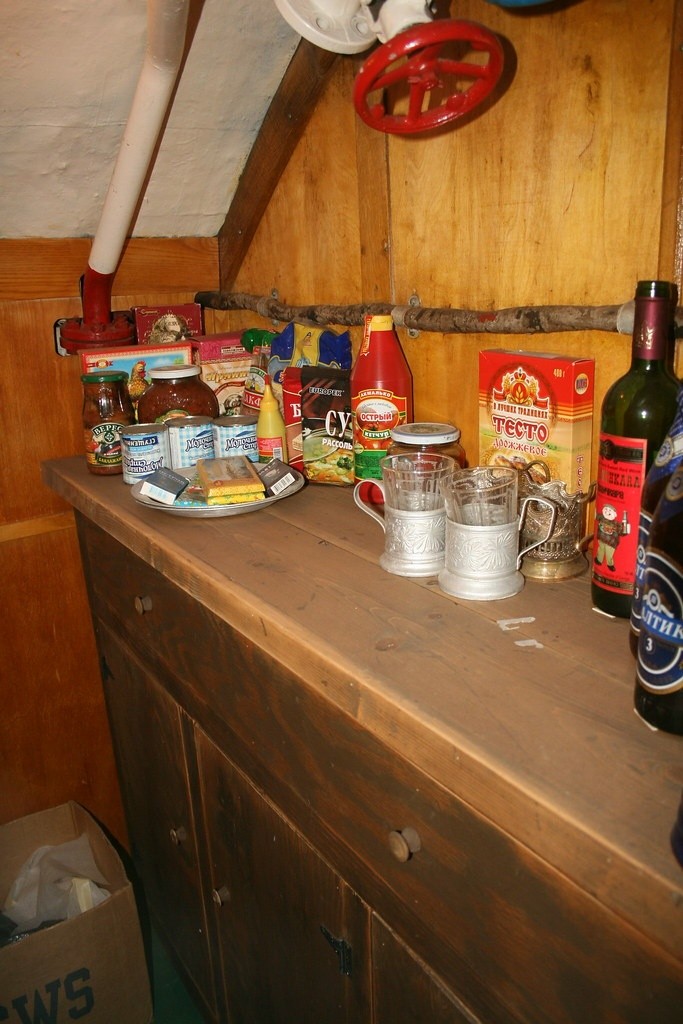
41 453 683 1024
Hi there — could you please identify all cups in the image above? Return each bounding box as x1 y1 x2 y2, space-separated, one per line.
438 495 556 601
438 465 519 524
378 452 454 510
353 478 456 578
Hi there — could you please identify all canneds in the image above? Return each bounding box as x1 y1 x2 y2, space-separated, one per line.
384 423 465 481
80 364 259 485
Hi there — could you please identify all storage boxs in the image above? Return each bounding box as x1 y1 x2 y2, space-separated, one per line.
131 303 203 344
78 340 192 423
283 365 356 486
191 330 252 418
0 800 154 1024
478 349 595 547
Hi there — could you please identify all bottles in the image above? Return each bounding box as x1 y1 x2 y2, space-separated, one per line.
350 313 413 504
634 459 683 735
629 389 683 661
256 375 288 466
592 280 678 618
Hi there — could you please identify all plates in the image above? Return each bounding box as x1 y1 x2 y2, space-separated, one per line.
129 463 306 518
